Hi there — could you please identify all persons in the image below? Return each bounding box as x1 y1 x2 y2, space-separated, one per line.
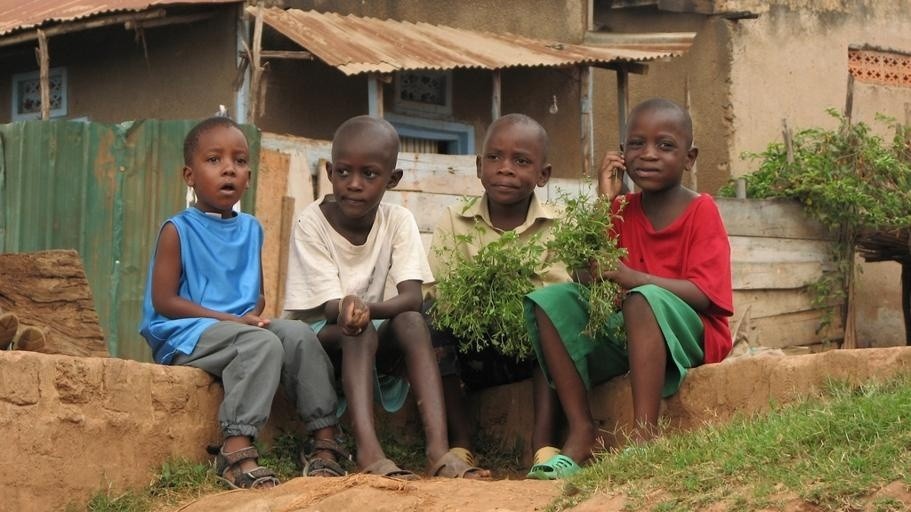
427 113 581 480
140 116 353 492
522 97 734 485
280 115 492 481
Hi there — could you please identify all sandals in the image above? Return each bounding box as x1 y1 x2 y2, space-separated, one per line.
304 437 354 477
205 440 281 489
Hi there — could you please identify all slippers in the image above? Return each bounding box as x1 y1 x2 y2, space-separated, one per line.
527 454 584 479
357 458 422 480
449 447 475 467
425 451 494 481
622 447 648 456
534 446 562 464
